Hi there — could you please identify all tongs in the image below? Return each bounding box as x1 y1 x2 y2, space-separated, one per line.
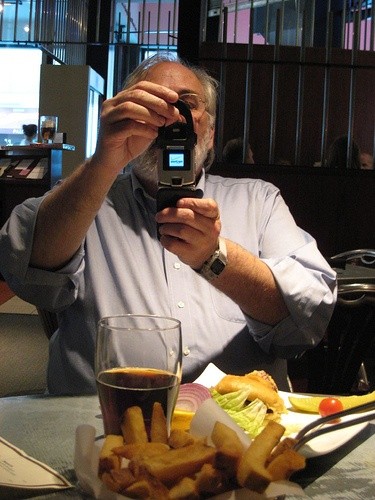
294 398 375 493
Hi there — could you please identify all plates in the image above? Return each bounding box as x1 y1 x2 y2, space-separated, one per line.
190 363 375 460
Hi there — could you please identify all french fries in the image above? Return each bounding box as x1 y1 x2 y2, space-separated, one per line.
99 401 306 500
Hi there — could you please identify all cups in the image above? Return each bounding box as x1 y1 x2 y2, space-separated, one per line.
95 314 183 444
39 115 58 144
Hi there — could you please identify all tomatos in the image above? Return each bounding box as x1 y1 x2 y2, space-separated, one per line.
318 398 344 423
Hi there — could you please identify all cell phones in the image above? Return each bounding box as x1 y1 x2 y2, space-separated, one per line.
157 99 204 241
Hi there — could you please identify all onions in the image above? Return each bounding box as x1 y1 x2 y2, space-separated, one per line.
175 382 212 412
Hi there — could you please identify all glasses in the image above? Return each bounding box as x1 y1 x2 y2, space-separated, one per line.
177 93 209 121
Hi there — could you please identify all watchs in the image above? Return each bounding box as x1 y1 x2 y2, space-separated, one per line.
190 235 231 282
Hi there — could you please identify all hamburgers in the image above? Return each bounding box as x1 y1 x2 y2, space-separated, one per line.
215 369 286 420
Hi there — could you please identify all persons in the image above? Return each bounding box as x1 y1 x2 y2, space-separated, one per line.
357 152 373 169
1 47 343 390
220 136 257 165
20 122 39 146
324 134 360 170
